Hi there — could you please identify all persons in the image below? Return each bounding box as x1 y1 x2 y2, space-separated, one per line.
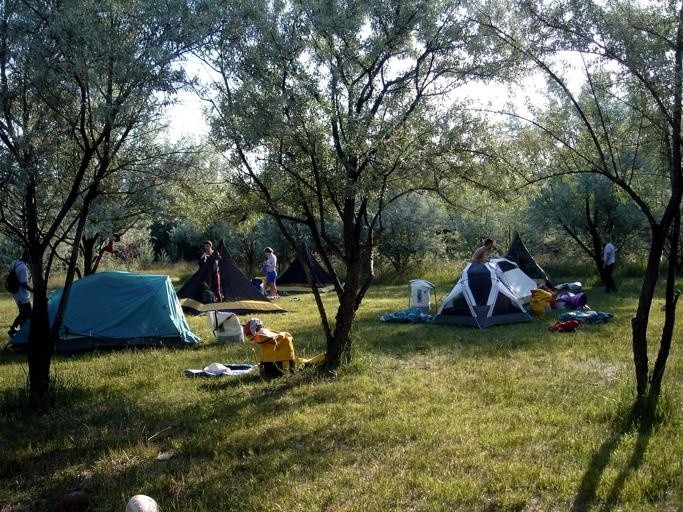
600 235 618 291
471 239 492 263
199 240 224 304
259 246 279 300
8 252 34 340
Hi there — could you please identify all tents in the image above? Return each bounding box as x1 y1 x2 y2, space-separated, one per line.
175 239 292 315
2 271 202 352
503 231 547 285
275 242 343 286
432 258 538 328
